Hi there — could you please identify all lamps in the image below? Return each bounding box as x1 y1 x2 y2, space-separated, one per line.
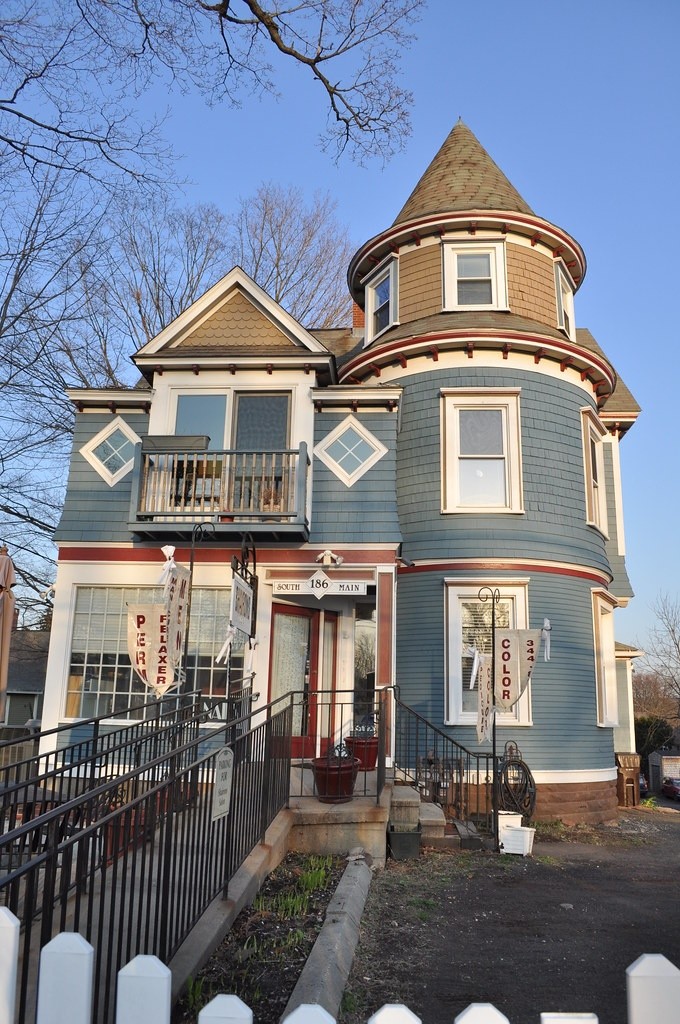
39 582 56 599
316 549 344 565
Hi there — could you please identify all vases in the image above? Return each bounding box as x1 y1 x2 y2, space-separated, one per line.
343 736 378 770
106 805 151 863
491 809 523 845
154 786 199 815
218 509 235 522
500 826 530 857
506 824 536 855
310 755 362 803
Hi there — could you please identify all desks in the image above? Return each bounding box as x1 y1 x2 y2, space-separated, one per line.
0 781 73 870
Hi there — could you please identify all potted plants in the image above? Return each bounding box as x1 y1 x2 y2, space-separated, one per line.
259 487 281 522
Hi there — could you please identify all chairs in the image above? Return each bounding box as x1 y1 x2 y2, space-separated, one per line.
35 773 121 853
